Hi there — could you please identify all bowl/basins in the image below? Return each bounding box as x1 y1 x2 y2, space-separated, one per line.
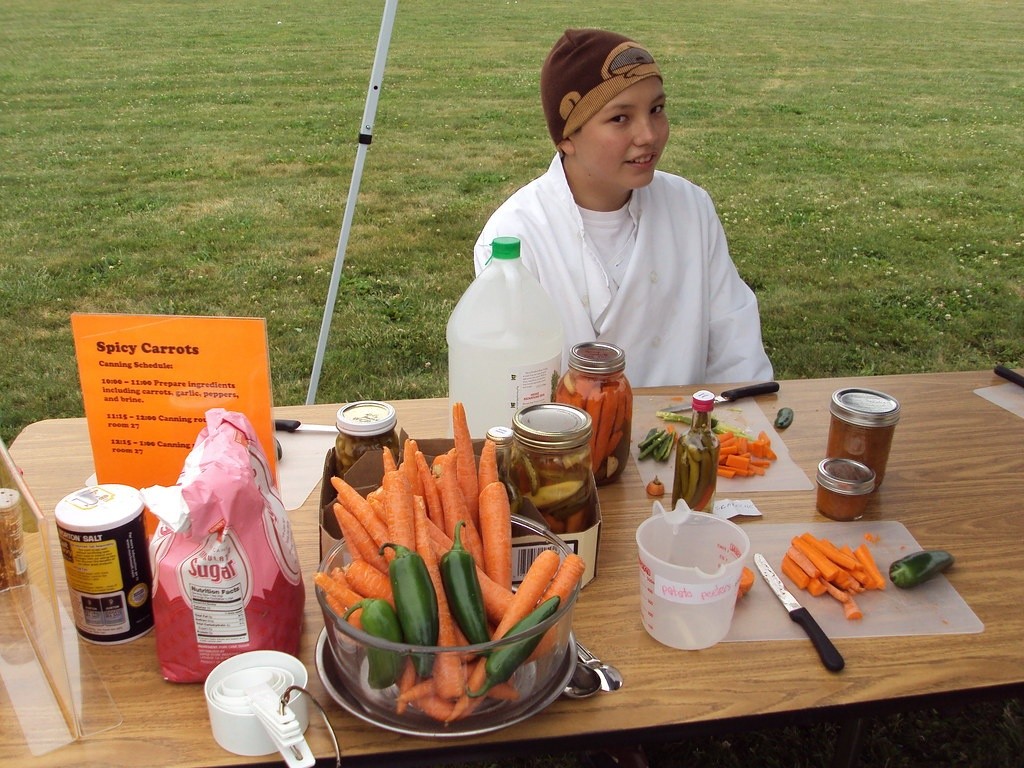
314 513 583 733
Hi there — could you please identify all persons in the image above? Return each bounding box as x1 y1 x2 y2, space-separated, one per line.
472 28 773 389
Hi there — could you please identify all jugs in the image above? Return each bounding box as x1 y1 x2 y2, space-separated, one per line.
635 498 752 651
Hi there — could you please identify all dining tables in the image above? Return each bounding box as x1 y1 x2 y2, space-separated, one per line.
0 368 1024 768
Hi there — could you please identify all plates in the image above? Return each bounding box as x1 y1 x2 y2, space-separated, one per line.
313 630 579 739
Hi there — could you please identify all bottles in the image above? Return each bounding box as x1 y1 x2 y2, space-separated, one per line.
486 427 522 515
446 237 562 438
671 390 721 515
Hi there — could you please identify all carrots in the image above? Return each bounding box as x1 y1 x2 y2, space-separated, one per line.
781 533 887 618
714 430 777 479
736 567 755 598
313 402 585 725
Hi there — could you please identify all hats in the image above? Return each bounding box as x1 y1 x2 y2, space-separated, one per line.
540 27 665 142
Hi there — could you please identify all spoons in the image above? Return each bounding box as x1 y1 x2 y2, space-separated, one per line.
562 641 624 698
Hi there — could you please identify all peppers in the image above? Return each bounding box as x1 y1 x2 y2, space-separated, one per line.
379 542 439 678
637 428 675 461
889 550 956 588
774 407 793 428
440 520 493 656
340 598 403 690
464 595 561 698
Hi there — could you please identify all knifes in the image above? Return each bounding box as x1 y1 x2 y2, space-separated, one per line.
660 381 780 412
274 419 339 432
753 553 845 672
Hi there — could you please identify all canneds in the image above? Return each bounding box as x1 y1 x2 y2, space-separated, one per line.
55 482 155 646
